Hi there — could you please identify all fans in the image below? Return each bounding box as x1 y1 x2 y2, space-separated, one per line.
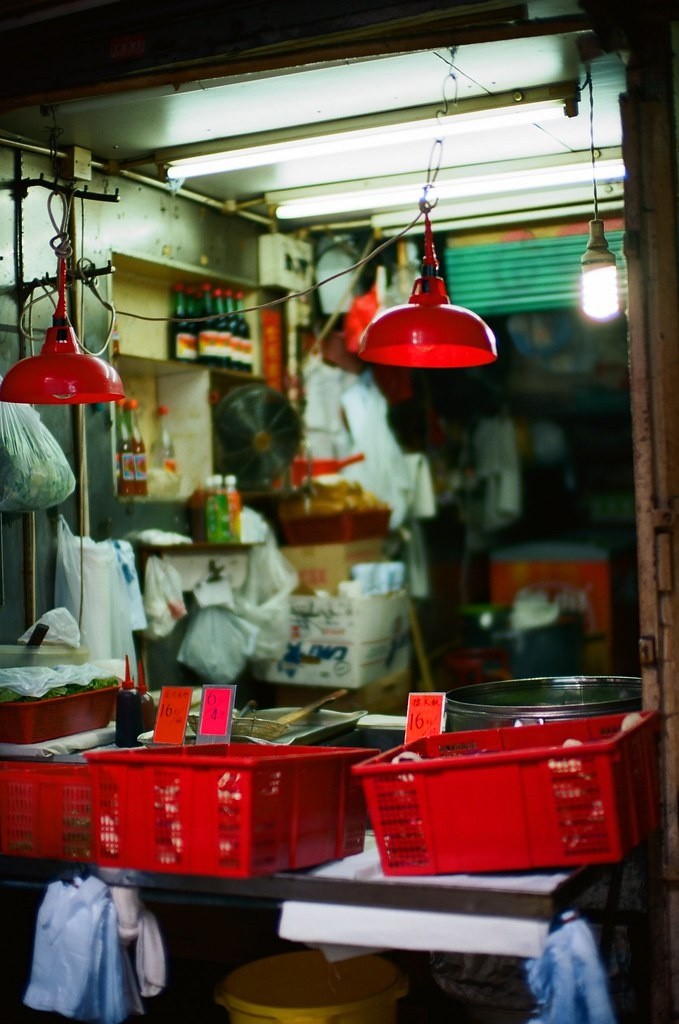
215 386 303 485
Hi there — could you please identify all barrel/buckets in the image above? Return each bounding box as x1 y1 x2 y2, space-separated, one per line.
213 951 410 1024
444 676 643 733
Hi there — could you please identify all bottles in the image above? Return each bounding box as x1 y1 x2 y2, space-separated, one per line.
188 473 241 542
167 282 253 373
150 406 178 497
115 395 147 497
115 655 155 749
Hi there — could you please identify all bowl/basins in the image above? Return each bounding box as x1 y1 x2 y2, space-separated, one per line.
0 643 93 670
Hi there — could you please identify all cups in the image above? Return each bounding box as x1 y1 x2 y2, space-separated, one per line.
337 560 403 597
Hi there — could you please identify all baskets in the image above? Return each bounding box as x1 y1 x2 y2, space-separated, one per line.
280 508 393 545
0 706 661 879
0 683 122 745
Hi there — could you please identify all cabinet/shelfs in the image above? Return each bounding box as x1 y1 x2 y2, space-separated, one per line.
106 239 265 503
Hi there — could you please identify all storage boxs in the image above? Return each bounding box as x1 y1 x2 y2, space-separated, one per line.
277 536 384 595
0 671 660 880
284 509 396 549
242 587 408 687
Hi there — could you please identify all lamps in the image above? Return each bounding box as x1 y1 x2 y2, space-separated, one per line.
0 190 128 404
358 211 498 369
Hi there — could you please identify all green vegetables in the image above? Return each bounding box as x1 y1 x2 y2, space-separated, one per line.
0 679 119 702
0 426 74 512
